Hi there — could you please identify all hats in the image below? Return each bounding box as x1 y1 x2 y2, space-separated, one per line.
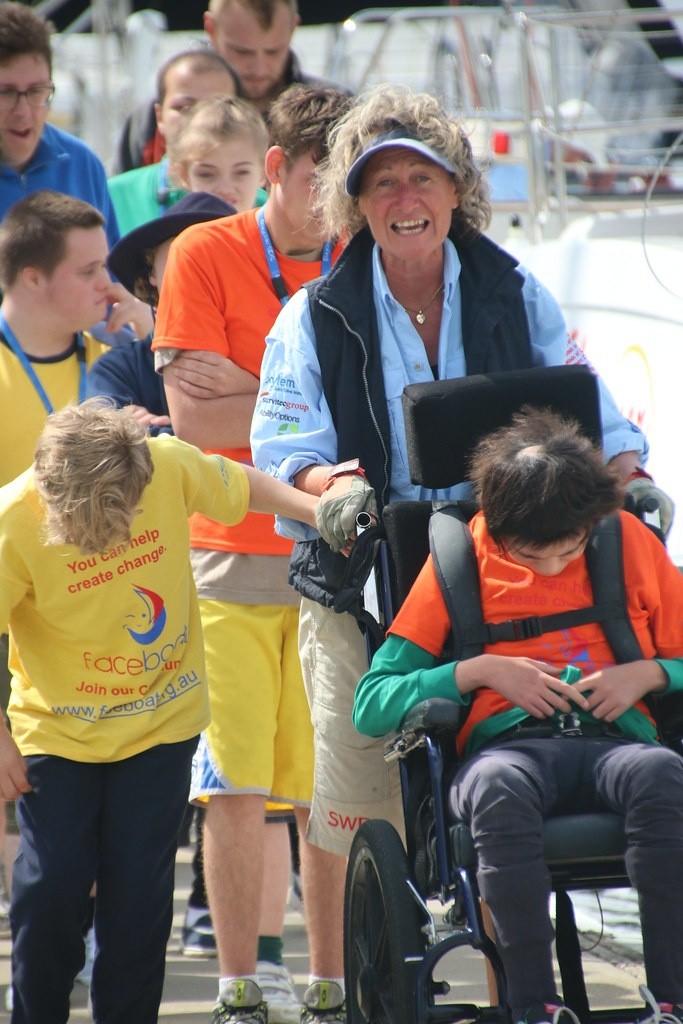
106 192 239 305
344 125 455 195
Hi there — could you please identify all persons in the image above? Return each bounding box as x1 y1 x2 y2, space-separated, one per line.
0 0 683 1024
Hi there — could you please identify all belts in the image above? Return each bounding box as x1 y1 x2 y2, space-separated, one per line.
503 709 597 736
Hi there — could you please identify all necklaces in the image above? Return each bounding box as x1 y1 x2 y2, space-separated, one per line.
397 283 445 325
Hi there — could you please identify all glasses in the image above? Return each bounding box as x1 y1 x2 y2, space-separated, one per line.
0 81 55 111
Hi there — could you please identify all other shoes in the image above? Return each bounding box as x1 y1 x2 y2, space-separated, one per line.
181 910 214 957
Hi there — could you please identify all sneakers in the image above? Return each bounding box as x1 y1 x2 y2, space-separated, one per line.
211 979 268 1024
636 985 683 1024
256 964 301 1024
517 997 579 1023
298 980 347 1024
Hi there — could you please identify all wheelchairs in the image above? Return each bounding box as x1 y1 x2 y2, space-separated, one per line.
343 365 683 1024
